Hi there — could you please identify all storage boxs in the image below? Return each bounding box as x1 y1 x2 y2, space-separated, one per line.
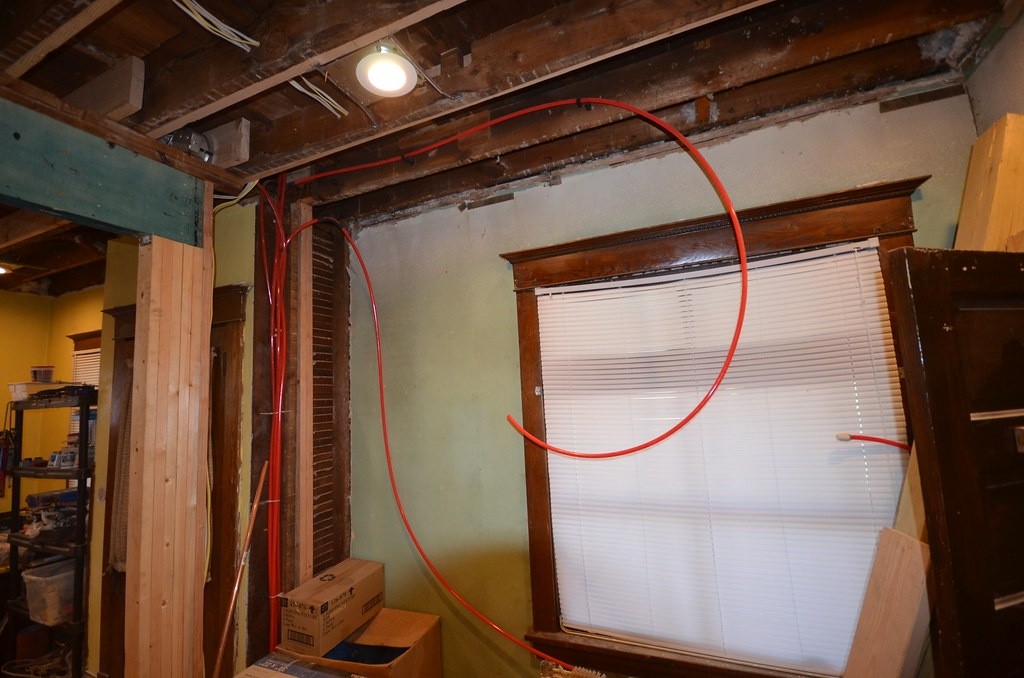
22 557 84 626
233 558 443 678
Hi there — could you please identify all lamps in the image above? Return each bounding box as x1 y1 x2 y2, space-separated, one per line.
356 40 418 97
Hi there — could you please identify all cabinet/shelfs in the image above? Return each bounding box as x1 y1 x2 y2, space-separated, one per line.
0 384 98 677
